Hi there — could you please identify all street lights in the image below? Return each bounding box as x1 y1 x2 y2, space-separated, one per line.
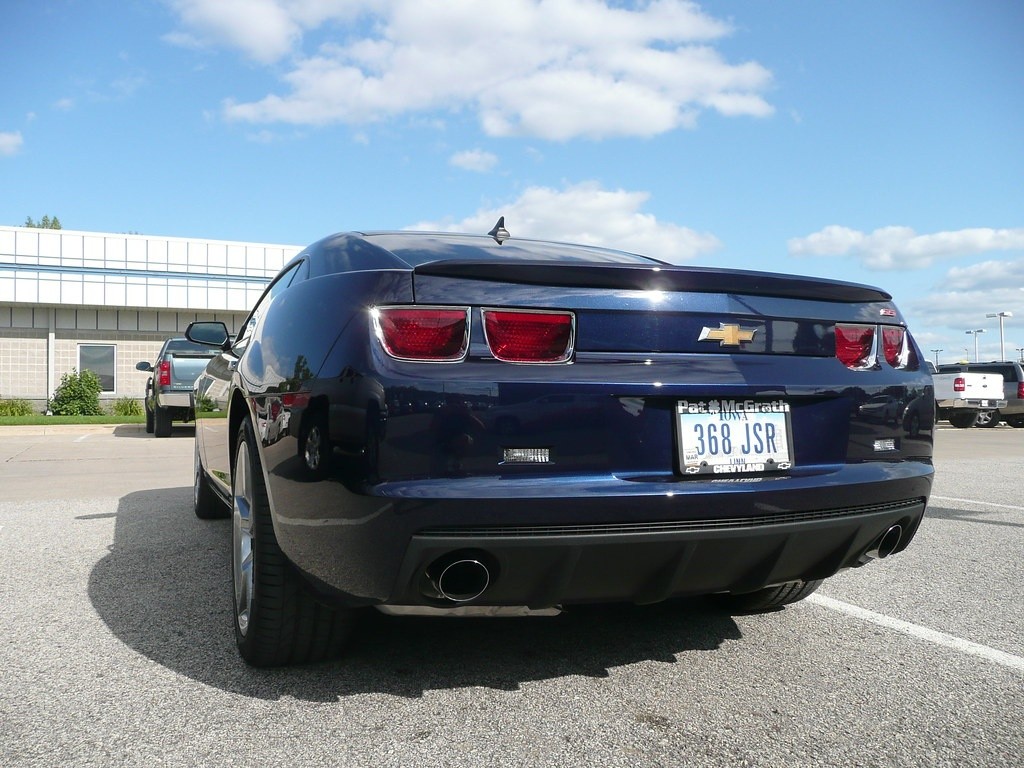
966 329 985 362
1016 348 1024 361
986 312 1012 363
930 349 943 363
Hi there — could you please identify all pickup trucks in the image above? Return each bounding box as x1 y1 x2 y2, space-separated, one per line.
135 337 236 438
925 359 1007 427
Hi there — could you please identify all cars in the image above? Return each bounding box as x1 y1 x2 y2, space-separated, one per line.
255 397 291 446
183 217 937 670
485 392 617 436
288 365 385 473
857 395 898 423
901 385 934 436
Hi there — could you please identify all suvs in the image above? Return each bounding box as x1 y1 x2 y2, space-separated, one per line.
938 361 1024 429
